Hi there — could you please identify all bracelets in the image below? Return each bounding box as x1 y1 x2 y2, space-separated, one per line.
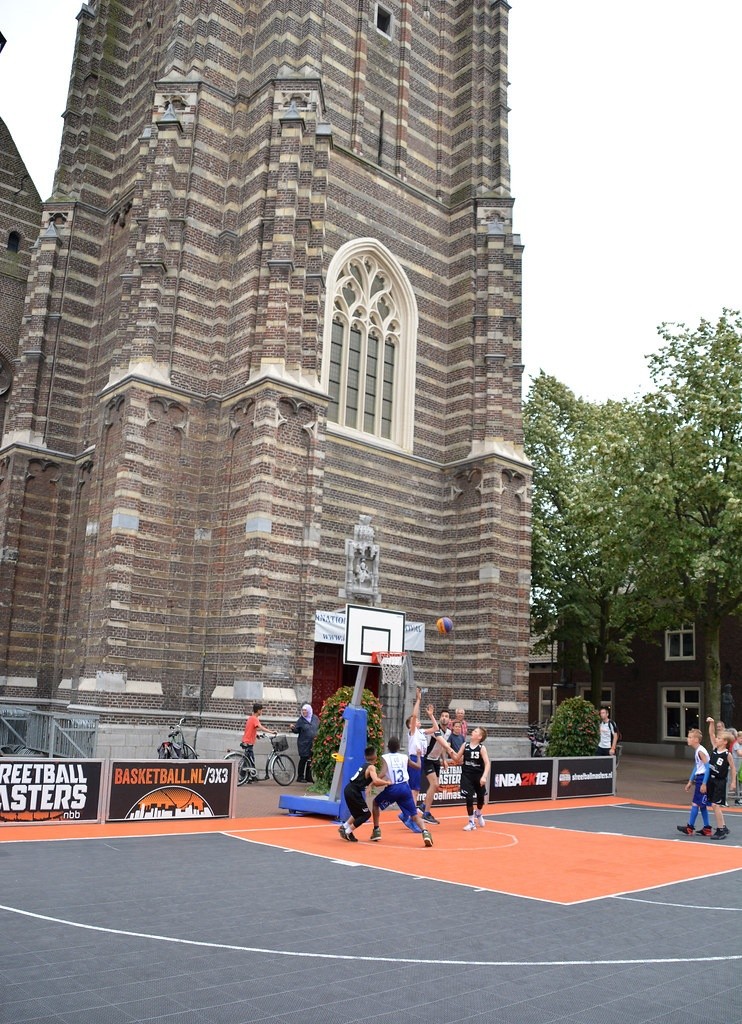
612 746 616 750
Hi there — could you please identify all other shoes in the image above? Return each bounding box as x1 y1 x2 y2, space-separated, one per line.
735 799 742 806
305 777 316 783
722 800 729 807
238 778 252 784
297 778 307 783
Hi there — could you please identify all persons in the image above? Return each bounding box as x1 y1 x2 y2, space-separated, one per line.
448 721 466 759
290 704 320 783
677 729 710 836
338 747 391 842
448 708 467 736
706 717 736 839
447 727 490 830
418 709 452 825
398 688 439 834
597 708 618 756
366 737 433 847
239 704 278 784
716 721 742 807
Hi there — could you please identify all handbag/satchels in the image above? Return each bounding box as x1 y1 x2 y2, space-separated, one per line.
157 741 166 759
169 742 182 759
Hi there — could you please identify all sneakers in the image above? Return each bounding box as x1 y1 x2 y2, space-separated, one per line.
397 812 409 824
405 818 422 833
696 825 712 836
711 828 726 840
344 828 358 842
677 824 695 836
723 825 729 834
423 832 433 847
338 825 349 840
417 803 426 814
421 813 440 824
463 822 476 831
370 829 382 840
473 810 485 826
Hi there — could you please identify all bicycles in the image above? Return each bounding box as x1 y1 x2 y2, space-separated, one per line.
222 730 296 786
527 718 554 757
162 717 197 759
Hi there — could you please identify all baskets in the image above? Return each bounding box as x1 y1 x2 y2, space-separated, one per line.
272 735 289 751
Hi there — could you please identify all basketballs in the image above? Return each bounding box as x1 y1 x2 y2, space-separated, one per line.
437 616 454 634
